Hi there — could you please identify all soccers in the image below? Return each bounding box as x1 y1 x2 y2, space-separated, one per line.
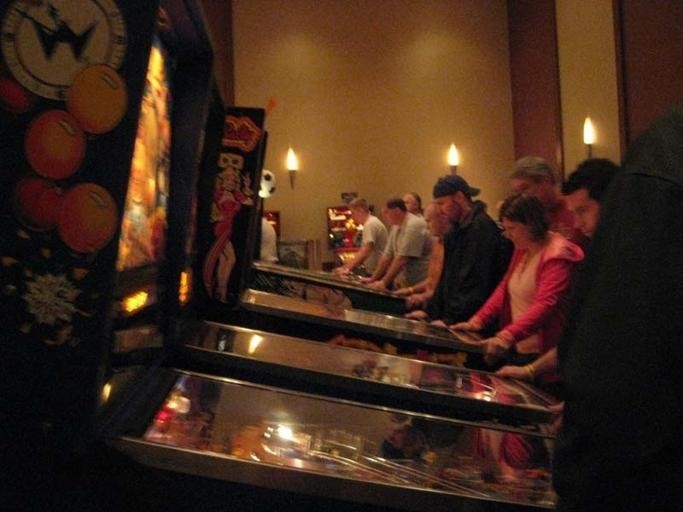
16 67 128 252
258 169 276 199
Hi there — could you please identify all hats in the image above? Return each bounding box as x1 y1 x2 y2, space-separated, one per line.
434 173 481 198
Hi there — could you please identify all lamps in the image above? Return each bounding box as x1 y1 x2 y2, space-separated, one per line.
283 144 298 192
582 115 596 158
446 143 461 179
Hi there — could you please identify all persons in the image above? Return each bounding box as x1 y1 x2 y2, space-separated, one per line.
551 106 683 512
